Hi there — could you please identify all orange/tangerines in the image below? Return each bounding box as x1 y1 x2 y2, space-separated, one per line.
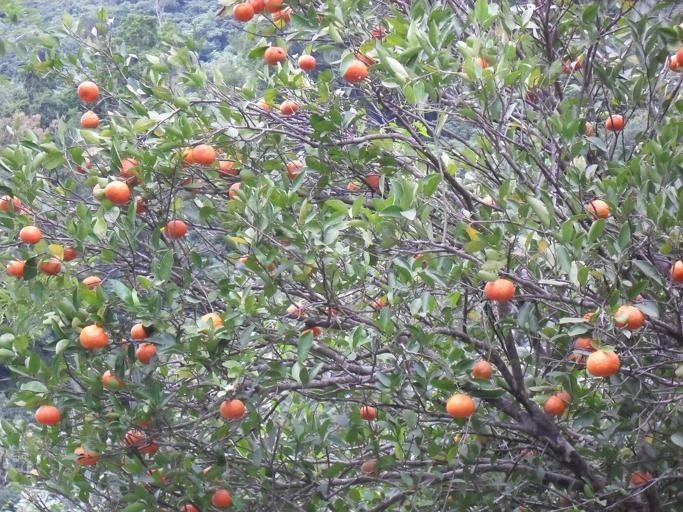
0 0 683 512
